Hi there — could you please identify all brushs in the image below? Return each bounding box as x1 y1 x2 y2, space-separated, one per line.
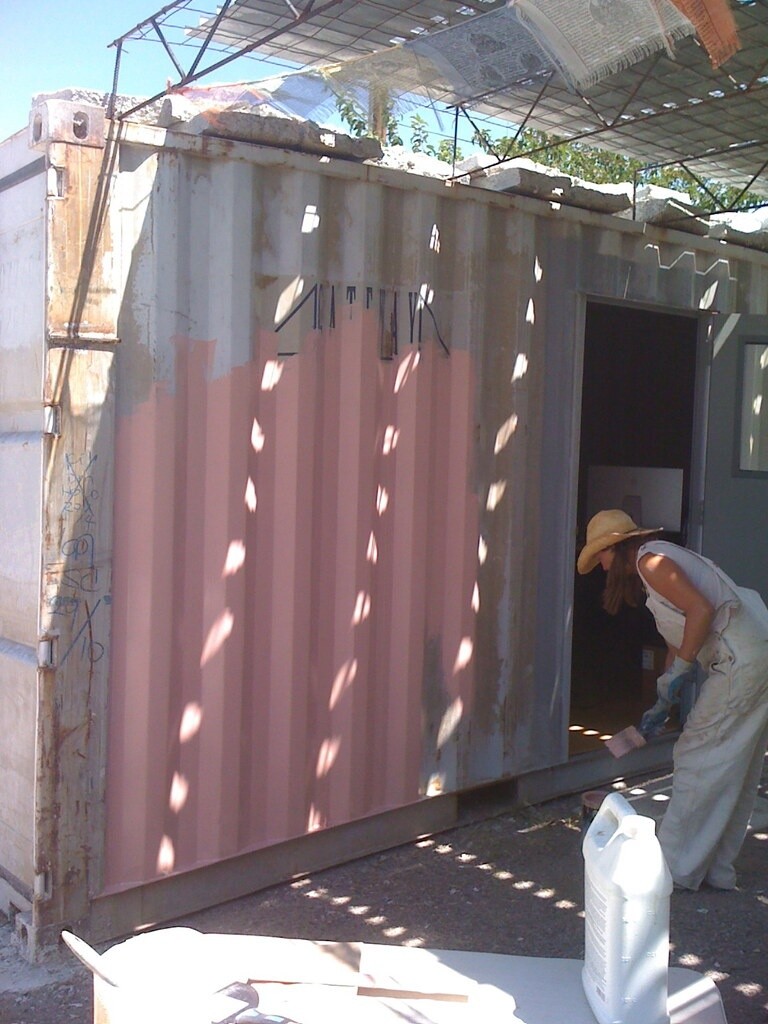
603 714 673 759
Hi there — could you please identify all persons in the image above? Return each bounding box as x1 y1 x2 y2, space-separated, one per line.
577 509 768 890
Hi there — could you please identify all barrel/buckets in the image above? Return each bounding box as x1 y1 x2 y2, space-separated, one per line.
581 790 609 830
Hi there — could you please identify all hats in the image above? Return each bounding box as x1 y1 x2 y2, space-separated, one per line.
577 510 663 575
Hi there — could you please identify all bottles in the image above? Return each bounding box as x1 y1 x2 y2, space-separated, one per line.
583 793 671 1024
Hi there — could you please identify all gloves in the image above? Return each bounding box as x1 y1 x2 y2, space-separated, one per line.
639 695 674 735
658 655 698 705
